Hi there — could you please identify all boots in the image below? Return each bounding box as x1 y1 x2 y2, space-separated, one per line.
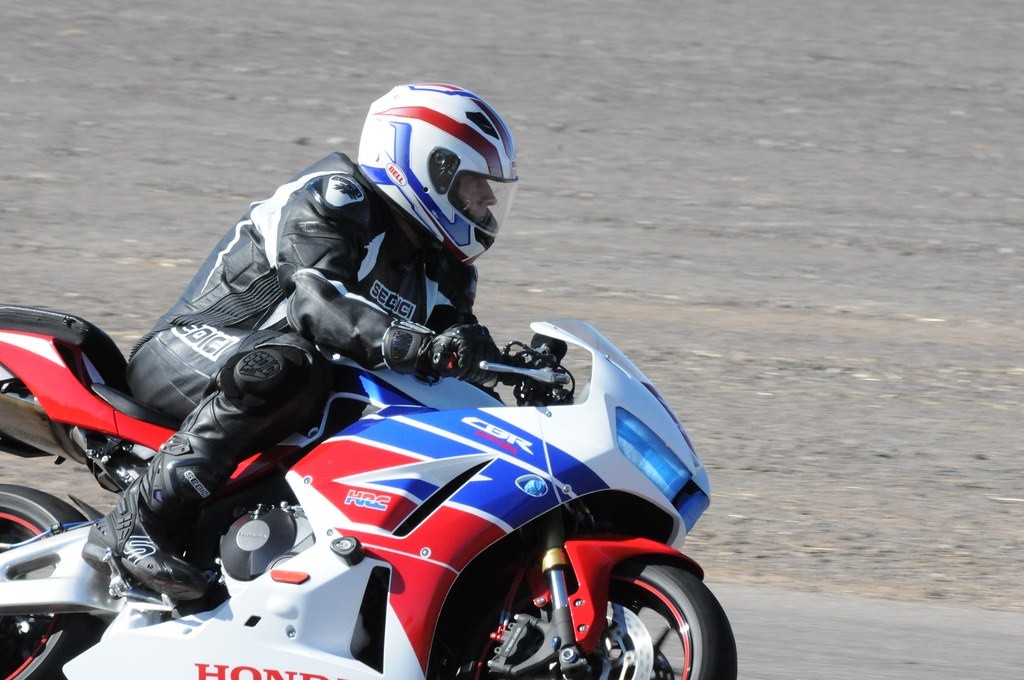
82 430 235 602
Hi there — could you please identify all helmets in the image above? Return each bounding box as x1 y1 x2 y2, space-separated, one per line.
357 82 518 265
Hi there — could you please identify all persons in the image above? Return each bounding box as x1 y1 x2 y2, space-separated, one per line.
81 83 519 599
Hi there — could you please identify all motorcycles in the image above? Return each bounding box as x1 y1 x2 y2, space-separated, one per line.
1 303 738 680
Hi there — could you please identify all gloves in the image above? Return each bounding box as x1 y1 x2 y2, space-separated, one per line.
381 323 500 386
